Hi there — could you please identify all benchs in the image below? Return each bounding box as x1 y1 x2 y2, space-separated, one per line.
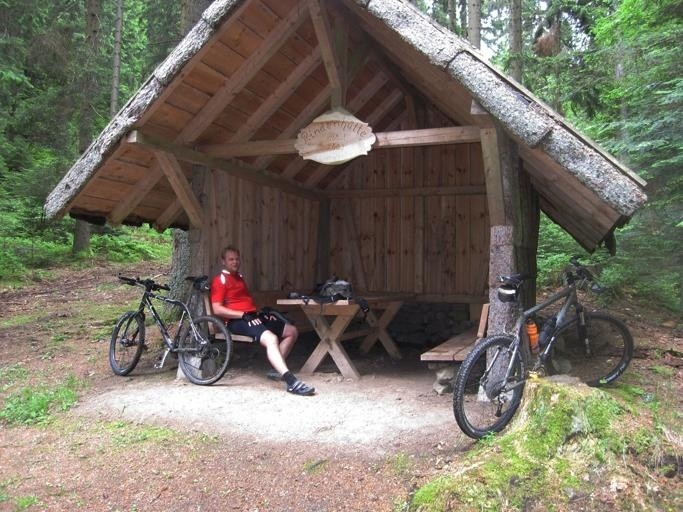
204 290 313 343
420 303 490 362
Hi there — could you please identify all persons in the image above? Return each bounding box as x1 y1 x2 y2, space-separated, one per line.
209 242 315 395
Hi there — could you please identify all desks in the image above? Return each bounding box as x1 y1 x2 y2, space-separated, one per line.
277 293 416 381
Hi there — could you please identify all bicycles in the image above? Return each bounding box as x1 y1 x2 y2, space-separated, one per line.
108 269 235 384
453 256 638 441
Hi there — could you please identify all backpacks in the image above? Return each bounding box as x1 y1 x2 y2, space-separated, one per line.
310 278 369 313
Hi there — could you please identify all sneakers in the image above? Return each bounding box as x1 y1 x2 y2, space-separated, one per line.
267 368 282 380
287 381 315 395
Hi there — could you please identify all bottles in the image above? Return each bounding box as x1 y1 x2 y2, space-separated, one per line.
525 318 540 354
